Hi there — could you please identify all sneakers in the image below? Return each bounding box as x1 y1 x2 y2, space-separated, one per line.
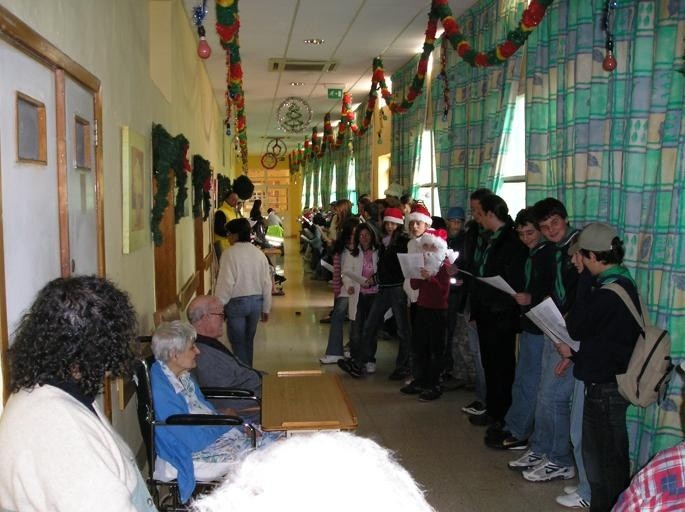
563 486 579 495
319 354 344 365
337 357 362 377
399 378 425 394
271 264 287 296
508 450 545 470
299 249 326 281
522 459 576 482
484 422 529 449
389 366 411 380
461 400 488 415
363 359 377 373
417 385 442 401
556 493 591 509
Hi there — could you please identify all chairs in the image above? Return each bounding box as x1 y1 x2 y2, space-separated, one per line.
145 354 261 418
133 360 260 511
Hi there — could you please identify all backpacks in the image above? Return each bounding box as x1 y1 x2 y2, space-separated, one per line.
599 281 674 408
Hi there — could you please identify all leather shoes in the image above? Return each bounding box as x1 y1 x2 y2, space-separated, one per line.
442 376 475 391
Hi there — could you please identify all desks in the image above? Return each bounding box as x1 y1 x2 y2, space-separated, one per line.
260 247 284 295
261 369 359 436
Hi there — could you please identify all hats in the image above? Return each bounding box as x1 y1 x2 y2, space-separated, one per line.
408 206 433 225
383 208 405 225
447 207 465 221
421 227 446 249
384 182 403 199
329 200 338 205
566 222 621 256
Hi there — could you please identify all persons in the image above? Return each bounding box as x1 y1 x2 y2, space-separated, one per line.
213 191 284 296
0 276 158 512
214 219 273 369
187 294 262 425
299 189 631 512
610 440 685 512
198 431 434 512
149 319 251 506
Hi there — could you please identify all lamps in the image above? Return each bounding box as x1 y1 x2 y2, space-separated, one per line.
226 124 231 136
197 25 211 59
442 109 449 122
603 40 617 71
377 132 382 144
